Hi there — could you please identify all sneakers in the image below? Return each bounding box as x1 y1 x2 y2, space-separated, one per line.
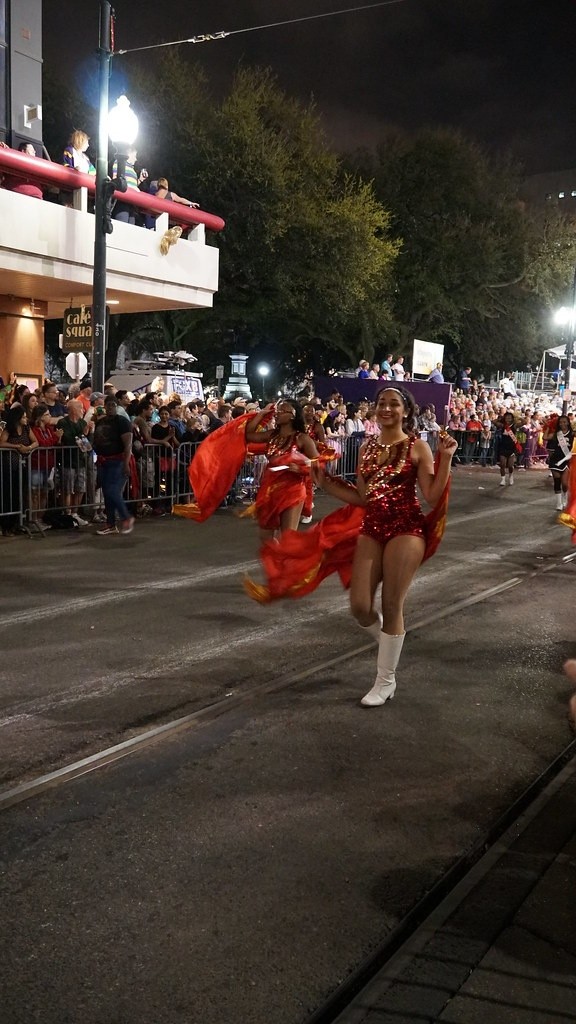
120 517 135 534
25 518 52 532
97 525 119 535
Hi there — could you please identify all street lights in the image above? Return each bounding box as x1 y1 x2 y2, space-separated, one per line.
88 96 141 394
258 365 270 409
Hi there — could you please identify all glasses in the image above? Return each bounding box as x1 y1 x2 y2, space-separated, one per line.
47 390 60 395
43 413 51 418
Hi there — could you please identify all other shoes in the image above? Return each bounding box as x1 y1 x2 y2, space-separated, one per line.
549 472 553 476
67 513 89 527
92 513 107 522
489 464 500 470
0 527 23 537
151 508 170 517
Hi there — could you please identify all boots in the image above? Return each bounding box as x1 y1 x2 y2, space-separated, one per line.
500 476 506 485
555 493 563 511
361 627 407 706
353 612 385 645
562 492 568 507
509 471 514 485
303 503 315 523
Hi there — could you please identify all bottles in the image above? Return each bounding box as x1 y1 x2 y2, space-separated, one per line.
75 435 86 452
81 434 92 451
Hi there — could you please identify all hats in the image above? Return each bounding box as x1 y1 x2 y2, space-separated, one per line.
246 403 260 411
234 396 248 406
206 397 220 405
90 392 108 401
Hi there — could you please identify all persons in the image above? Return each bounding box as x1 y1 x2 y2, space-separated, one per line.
4 143 43 199
113 145 149 224
456 367 472 394
243 398 325 547
491 412 527 486
145 178 199 232
58 130 97 209
310 386 458 706
543 415 576 511
355 354 410 381
426 363 444 383
0 372 576 534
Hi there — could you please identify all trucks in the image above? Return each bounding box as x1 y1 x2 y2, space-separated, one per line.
104 369 205 407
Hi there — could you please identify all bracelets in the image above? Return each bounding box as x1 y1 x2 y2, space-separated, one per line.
139 178 143 183
